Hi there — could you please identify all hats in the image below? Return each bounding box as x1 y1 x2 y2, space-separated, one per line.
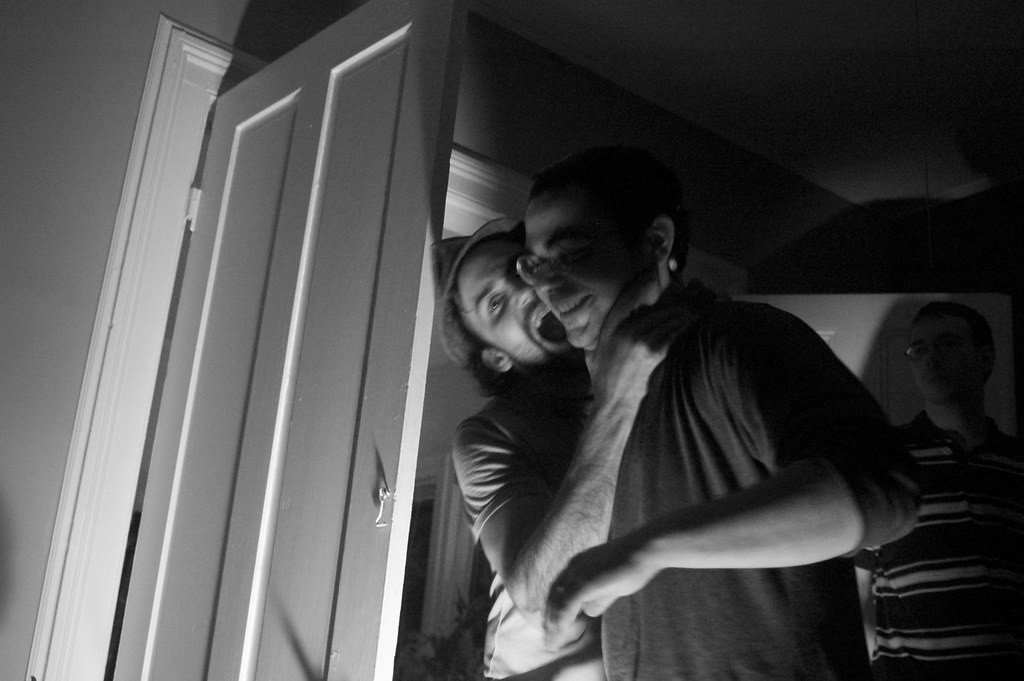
430 215 526 369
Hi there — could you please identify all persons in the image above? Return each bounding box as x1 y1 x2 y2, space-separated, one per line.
852 297 1024 681
433 217 702 681
522 143 924 681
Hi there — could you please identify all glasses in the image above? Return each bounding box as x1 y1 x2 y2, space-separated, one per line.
903 335 954 362
515 247 575 286
